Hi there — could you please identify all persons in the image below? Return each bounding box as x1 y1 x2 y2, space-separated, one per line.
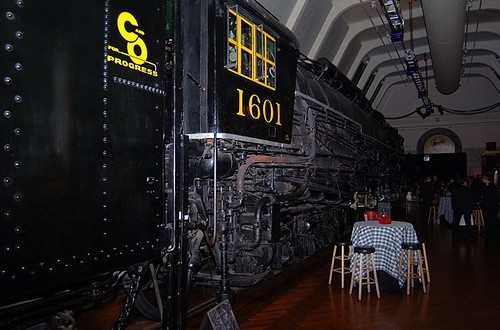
412 182 434 219
452 173 500 239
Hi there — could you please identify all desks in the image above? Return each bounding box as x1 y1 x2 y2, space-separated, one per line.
349 220 419 293
437 196 453 224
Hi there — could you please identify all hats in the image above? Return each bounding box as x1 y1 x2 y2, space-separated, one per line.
481 176 491 180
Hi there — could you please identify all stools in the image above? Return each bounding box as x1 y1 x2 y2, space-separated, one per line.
428 203 438 225
349 246 380 299
472 208 484 230
398 238 430 295
328 240 352 288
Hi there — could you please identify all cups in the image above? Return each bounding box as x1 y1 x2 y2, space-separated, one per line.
378 203 390 224
